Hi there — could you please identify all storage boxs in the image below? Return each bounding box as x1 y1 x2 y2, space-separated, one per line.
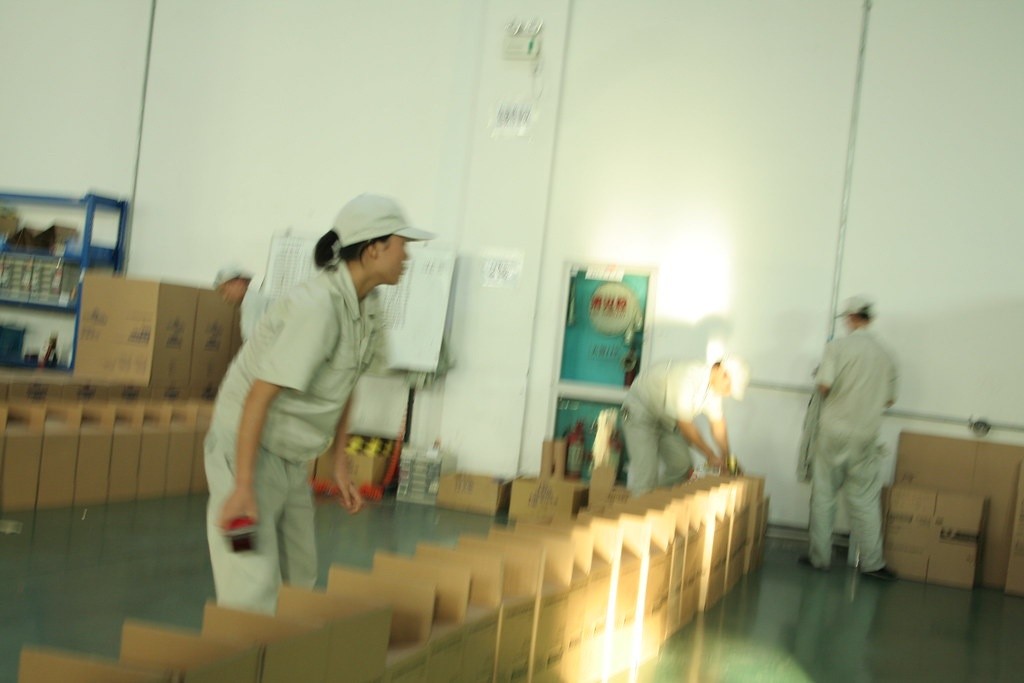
0 224 1024 683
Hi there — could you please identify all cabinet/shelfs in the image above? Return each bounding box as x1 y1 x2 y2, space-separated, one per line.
0 191 128 372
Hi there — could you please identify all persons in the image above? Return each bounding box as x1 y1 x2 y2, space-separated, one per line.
203 192 436 618
801 295 900 579
619 342 746 504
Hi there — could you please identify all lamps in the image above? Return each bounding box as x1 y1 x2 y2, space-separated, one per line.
501 17 543 60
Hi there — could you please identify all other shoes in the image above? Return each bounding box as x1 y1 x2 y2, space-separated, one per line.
799 555 828 570
860 566 896 582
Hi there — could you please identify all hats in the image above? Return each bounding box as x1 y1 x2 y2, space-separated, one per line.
831 296 876 318
334 192 436 248
213 266 253 290
721 354 750 401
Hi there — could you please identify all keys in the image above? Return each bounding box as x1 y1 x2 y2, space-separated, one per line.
619 405 630 422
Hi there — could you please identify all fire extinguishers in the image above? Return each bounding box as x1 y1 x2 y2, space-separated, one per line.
559 418 586 483
589 417 622 482
